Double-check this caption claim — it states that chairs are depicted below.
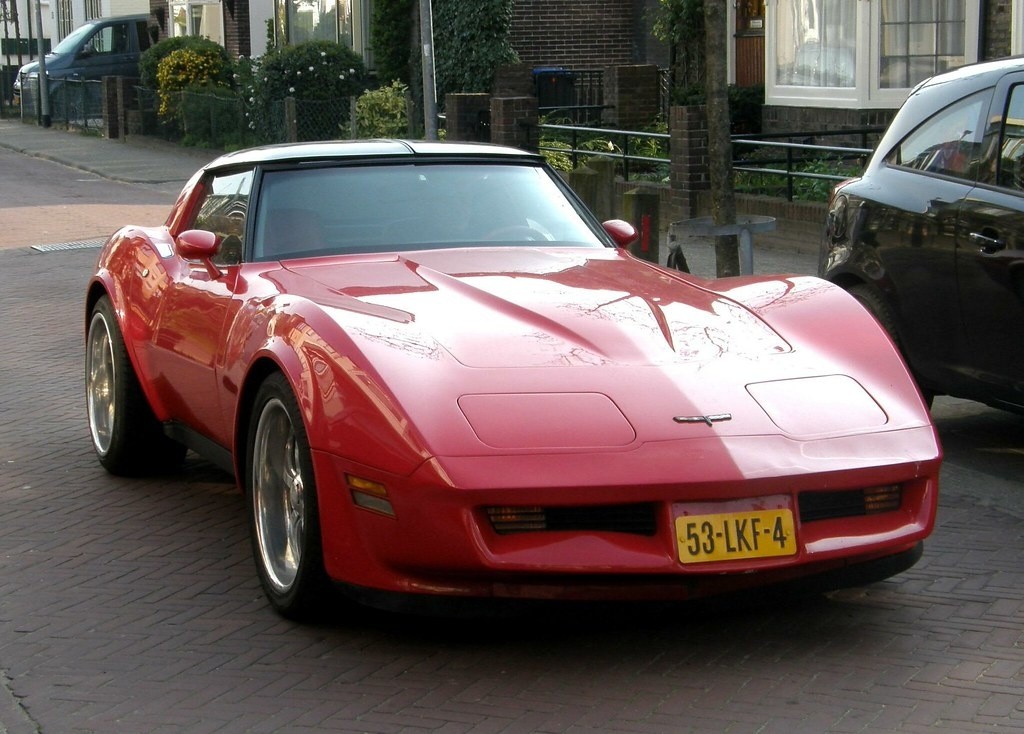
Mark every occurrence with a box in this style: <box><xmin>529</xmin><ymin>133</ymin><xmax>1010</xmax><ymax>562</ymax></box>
<box><xmin>431</xmin><ymin>178</ymin><xmax>530</xmax><ymax>240</ymax></box>
<box><xmin>263</xmin><ymin>208</ymin><xmax>324</xmax><ymax>252</ymax></box>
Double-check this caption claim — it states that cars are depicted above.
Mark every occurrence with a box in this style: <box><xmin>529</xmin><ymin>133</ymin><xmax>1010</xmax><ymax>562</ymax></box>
<box><xmin>817</xmin><ymin>54</ymin><xmax>1024</xmax><ymax>414</ymax></box>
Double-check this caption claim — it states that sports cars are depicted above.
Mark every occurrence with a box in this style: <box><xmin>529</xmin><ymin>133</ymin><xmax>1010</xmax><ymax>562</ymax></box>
<box><xmin>85</xmin><ymin>139</ymin><xmax>944</xmax><ymax>620</ymax></box>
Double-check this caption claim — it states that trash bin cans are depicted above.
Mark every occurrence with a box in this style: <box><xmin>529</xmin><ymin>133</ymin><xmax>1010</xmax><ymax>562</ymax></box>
<box><xmin>534</xmin><ymin>68</ymin><xmax>576</xmax><ymax>124</ymax></box>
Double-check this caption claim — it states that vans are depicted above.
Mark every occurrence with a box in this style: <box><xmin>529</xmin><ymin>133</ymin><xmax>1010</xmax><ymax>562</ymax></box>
<box><xmin>13</xmin><ymin>13</ymin><xmax>151</xmax><ymax>116</ymax></box>
